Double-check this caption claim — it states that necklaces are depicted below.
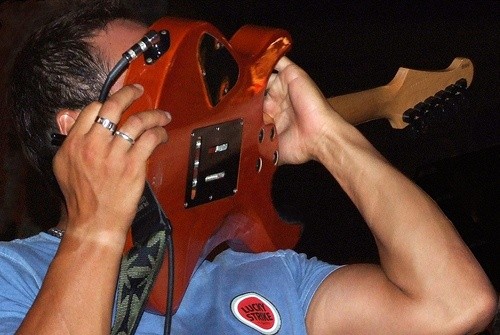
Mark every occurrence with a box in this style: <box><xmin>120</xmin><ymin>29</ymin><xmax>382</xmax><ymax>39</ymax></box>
<box><xmin>47</xmin><ymin>226</ymin><xmax>66</xmax><ymax>238</ymax></box>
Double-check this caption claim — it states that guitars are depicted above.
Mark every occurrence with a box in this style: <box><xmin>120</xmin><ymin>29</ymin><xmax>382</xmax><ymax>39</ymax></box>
<box><xmin>114</xmin><ymin>17</ymin><xmax>473</xmax><ymax>317</ymax></box>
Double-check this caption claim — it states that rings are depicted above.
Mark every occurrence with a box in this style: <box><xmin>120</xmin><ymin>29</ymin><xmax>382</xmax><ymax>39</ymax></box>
<box><xmin>95</xmin><ymin>116</ymin><xmax>117</xmax><ymax>133</ymax></box>
<box><xmin>114</xmin><ymin>130</ymin><xmax>135</xmax><ymax>145</ymax></box>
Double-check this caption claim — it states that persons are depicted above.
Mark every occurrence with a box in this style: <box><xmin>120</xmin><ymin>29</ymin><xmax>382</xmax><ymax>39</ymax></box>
<box><xmin>0</xmin><ymin>5</ymin><xmax>500</xmax><ymax>335</ymax></box>
